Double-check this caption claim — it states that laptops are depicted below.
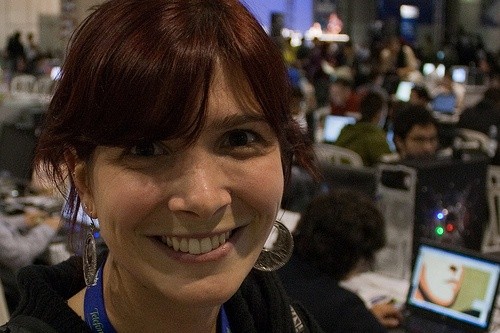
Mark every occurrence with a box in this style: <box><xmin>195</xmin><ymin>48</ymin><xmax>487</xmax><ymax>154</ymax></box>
<box><xmin>49</xmin><ymin>186</ymin><xmax>101</xmax><ymax>244</ymax></box>
<box><xmin>321</xmin><ymin>115</ymin><xmax>356</xmax><ymax>144</ymax></box>
<box><xmin>385</xmin><ymin>237</ymin><xmax>500</xmax><ymax>333</ymax></box>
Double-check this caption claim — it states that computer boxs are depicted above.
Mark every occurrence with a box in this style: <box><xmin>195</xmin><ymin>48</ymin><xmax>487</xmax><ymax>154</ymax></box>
<box><xmin>371</xmin><ymin>153</ymin><xmax>488</xmax><ymax>279</ymax></box>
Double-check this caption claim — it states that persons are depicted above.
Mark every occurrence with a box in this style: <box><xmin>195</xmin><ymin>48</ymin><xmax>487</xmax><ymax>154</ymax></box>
<box><xmin>1</xmin><ymin>204</ymin><xmax>64</xmax><ymax>270</ymax></box>
<box><xmin>3</xmin><ymin>0</ymin><xmax>386</xmax><ymax>331</ymax></box>
<box><xmin>292</xmin><ymin>192</ymin><xmax>404</xmax><ymax>330</ymax></box>
<box><xmin>5</xmin><ymin>29</ymin><xmax>56</xmax><ymax>76</ymax></box>
<box><xmin>280</xmin><ymin>32</ymin><xmax>499</xmax><ymax>166</ymax></box>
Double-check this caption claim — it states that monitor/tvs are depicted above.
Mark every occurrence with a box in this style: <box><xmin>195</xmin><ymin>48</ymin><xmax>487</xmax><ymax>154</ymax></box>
<box><xmin>449</xmin><ymin>65</ymin><xmax>469</xmax><ymax>85</ymax></box>
<box><xmin>395</xmin><ymin>81</ymin><xmax>416</xmax><ymax>103</ymax></box>
